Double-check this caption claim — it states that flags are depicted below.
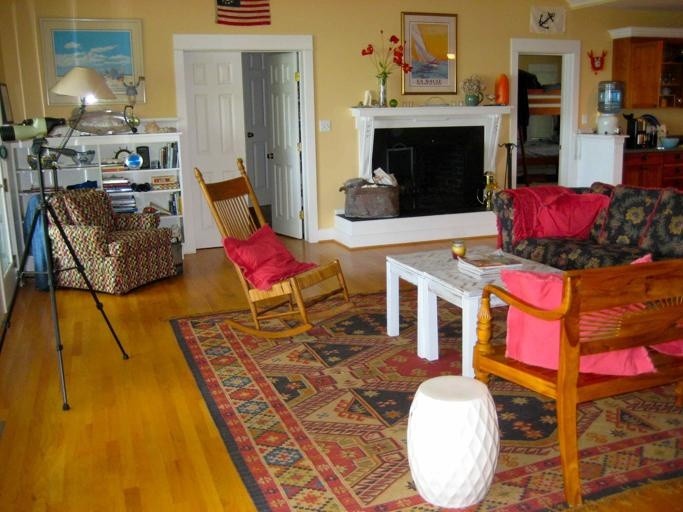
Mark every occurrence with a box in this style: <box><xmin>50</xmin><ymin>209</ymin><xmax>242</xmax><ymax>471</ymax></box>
<box><xmin>216</xmin><ymin>1</ymin><xmax>272</xmax><ymax>26</ymax></box>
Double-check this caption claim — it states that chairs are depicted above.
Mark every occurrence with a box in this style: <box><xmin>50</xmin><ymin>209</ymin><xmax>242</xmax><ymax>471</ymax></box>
<box><xmin>194</xmin><ymin>159</ymin><xmax>356</xmax><ymax>341</ymax></box>
<box><xmin>24</xmin><ymin>188</ymin><xmax>178</xmax><ymax>296</ymax></box>
<box><xmin>473</xmin><ymin>258</ymin><xmax>683</xmax><ymax>507</ymax></box>
<box><xmin>494</xmin><ymin>183</ymin><xmax>683</xmax><ymax>271</ymax></box>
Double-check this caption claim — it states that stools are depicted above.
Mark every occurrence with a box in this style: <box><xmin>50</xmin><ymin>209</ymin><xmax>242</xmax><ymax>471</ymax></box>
<box><xmin>406</xmin><ymin>375</ymin><xmax>500</xmax><ymax>511</ymax></box>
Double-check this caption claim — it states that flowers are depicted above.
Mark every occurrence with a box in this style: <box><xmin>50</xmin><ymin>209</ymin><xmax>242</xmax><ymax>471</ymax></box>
<box><xmin>361</xmin><ymin>28</ymin><xmax>413</xmax><ymax>85</ymax></box>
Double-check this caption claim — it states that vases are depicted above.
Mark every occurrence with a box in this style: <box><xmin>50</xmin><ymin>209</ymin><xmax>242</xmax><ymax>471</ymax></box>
<box><xmin>379</xmin><ymin>72</ymin><xmax>386</xmax><ymax>106</ymax></box>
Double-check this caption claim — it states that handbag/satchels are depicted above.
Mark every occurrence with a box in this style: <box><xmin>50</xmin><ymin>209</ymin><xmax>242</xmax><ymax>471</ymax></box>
<box><xmin>341</xmin><ymin>176</ymin><xmax>401</xmax><ymax>220</ymax></box>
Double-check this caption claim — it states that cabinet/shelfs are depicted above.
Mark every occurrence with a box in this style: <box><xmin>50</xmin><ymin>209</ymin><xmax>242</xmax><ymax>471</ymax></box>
<box><xmin>612</xmin><ymin>36</ymin><xmax>662</xmax><ymax>109</ymax></box>
<box><xmin>659</xmin><ymin>38</ymin><xmax>683</xmax><ymax>109</ymax></box>
<box><xmin>10</xmin><ymin>132</ymin><xmax>186</xmax><ymax>273</ymax></box>
<box><xmin>623</xmin><ymin>151</ymin><xmax>683</xmax><ymax>190</ymax></box>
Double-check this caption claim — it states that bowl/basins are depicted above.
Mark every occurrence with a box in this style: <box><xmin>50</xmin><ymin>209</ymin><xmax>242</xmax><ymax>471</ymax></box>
<box><xmin>659</xmin><ymin>137</ymin><xmax>680</xmax><ymax>150</ymax></box>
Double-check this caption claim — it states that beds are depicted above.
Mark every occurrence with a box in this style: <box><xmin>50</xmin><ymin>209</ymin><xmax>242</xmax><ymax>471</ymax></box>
<box><xmin>517</xmin><ymin>87</ymin><xmax>561</xmax><ymax>183</ymax></box>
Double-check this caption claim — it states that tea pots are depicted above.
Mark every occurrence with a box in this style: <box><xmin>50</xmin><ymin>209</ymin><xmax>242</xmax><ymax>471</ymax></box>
<box><xmin>465</xmin><ymin>92</ymin><xmax>483</xmax><ymax>106</ymax></box>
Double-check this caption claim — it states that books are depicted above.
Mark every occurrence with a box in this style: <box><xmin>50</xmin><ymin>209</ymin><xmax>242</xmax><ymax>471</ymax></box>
<box><xmin>456</xmin><ymin>247</ymin><xmax>523</xmax><ymax>283</ymax></box>
<box><xmin>101</xmin><ymin>158</ymin><xmax>139</xmax><ymax>214</ymax></box>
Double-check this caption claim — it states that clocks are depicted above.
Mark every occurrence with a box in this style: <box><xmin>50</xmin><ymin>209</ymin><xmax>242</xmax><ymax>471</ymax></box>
<box><xmin>111</xmin><ymin>145</ymin><xmax>133</xmax><ymax>166</ymax></box>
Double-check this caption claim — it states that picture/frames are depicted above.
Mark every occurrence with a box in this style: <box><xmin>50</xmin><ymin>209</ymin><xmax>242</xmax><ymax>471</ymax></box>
<box><xmin>40</xmin><ymin>17</ymin><xmax>146</xmax><ymax>104</ymax></box>
<box><xmin>401</xmin><ymin>12</ymin><xmax>457</xmax><ymax>95</ymax></box>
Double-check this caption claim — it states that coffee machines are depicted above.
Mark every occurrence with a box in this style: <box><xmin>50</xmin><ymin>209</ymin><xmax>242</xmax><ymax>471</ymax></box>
<box><xmin>624</xmin><ymin>110</ymin><xmax>658</xmax><ymax>151</ymax></box>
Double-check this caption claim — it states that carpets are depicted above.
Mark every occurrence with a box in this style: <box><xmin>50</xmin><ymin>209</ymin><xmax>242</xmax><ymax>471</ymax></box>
<box><xmin>170</xmin><ymin>287</ymin><xmax>681</xmax><ymax>512</ymax></box>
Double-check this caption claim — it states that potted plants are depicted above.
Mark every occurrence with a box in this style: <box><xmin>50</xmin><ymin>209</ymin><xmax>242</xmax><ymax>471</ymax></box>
<box><xmin>459</xmin><ymin>72</ymin><xmax>487</xmax><ymax>106</ymax></box>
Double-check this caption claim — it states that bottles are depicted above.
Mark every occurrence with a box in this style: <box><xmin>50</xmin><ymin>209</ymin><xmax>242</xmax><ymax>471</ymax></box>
<box><xmin>495</xmin><ymin>73</ymin><xmax>509</xmax><ymax>104</ymax></box>
<box><xmin>451</xmin><ymin>240</ymin><xmax>466</xmax><ymax>259</ymax></box>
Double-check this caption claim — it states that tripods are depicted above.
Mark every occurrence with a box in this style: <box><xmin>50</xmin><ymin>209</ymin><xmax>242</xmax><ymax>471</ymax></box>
<box><xmin>0</xmin><ymin>144</ymin><xmax>130</xmax><ymax>412</ymax></box>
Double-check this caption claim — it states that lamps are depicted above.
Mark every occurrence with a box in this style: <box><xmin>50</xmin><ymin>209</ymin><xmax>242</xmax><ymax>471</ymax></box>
<box><xmin>44</xmin><ymin>68</ymin><xmax>117</xmax><ymax>192</ymax></box>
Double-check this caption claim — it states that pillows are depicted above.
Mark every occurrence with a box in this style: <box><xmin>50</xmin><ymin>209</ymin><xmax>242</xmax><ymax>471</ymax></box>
<box><xmin>221</xmin><ymin>228</ymin><xmax>319</xmax><ymax>291</ymax></box>
<box><xmin>499</xmin><ymin>253</ymin><xmax>683</xmax><ymax>376</ymax></box>
<box><xmin>521</xmin><ymin>183</ymin><xmax>683</xmax><ymax>258</ymax></box>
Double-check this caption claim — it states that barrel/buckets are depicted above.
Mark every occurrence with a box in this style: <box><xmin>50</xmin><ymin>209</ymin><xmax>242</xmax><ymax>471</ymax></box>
<box><xmin>597</xmin><ymin>80</ymin><xmax>623</xmax><ymax>114</ymax></box>
